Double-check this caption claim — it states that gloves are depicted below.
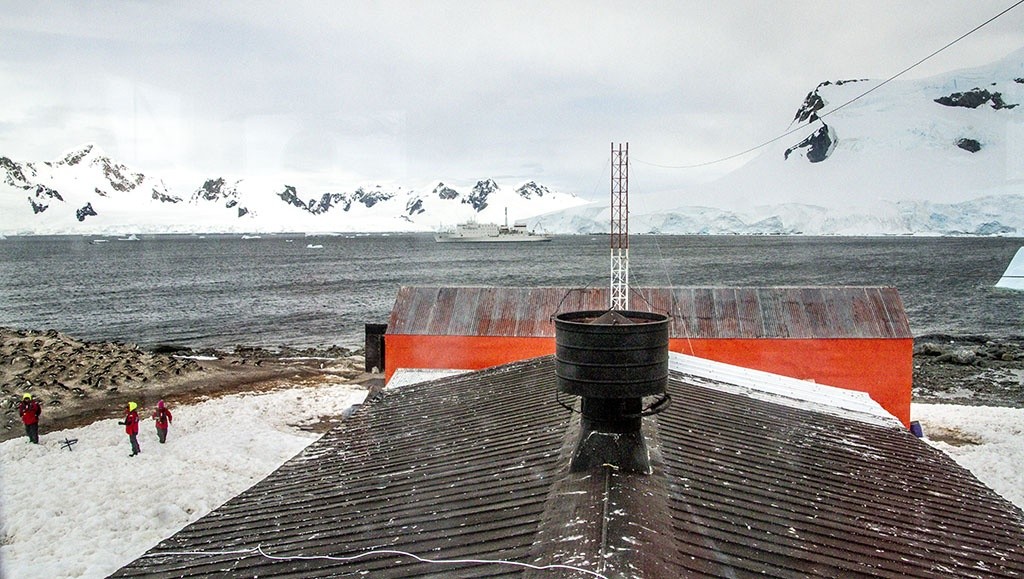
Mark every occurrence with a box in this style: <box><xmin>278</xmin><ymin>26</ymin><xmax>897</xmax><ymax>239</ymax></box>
<box><xmin>118</xmin><ymin>422</ymin><xmax>123</xmax><ymax>426</ymax></box>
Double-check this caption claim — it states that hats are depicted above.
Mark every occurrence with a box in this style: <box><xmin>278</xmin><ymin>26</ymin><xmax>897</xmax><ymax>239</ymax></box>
<box><xmin>159</xmin><ymin>400</ymin><xmax>164</xmax><ymax>408</ymax></box>
<box><xmin>128</xmin><ymin>402</ymin><xmax>137</xmax><ymax>411</ymax></box>
<box><xmin>22</xmin><ymin>393</ymin><xmax>31</xmax><ymax>400</ymax></box>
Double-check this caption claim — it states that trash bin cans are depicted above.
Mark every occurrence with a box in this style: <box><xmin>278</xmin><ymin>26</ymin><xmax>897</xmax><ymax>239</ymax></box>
<box><xmin>910</xmin><ymin>421</ymin><xmax>923</xmax><ymax>438</ymax></box>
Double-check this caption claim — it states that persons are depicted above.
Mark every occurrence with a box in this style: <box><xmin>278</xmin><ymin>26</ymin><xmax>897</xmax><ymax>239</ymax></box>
<box><xmin>152</xmin><ymin>401</ymin><xmax>173</xmax><ymax>443</ymax></box>
<box><xmin>18</xmin><ymin>393</ymin><xmax>42</xmax><ymax>444</ymax></box>
<box><xmin>118</xmin><ymin>402</ymin><xmax>141</xmax><ymax>457</ymax></box>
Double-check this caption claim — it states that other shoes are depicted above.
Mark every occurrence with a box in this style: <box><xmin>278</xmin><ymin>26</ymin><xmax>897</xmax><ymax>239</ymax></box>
<box><xmin>129</xmin><ymin>452</ymin><xmax>137</xmax><ymax>457</ymax></box>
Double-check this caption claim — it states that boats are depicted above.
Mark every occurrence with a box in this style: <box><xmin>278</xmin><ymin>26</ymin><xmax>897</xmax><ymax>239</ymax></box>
<box><xmin>435</xmin><ymin>205</ymin><xmax>552</xmax><ymax>243</ymax></box>
